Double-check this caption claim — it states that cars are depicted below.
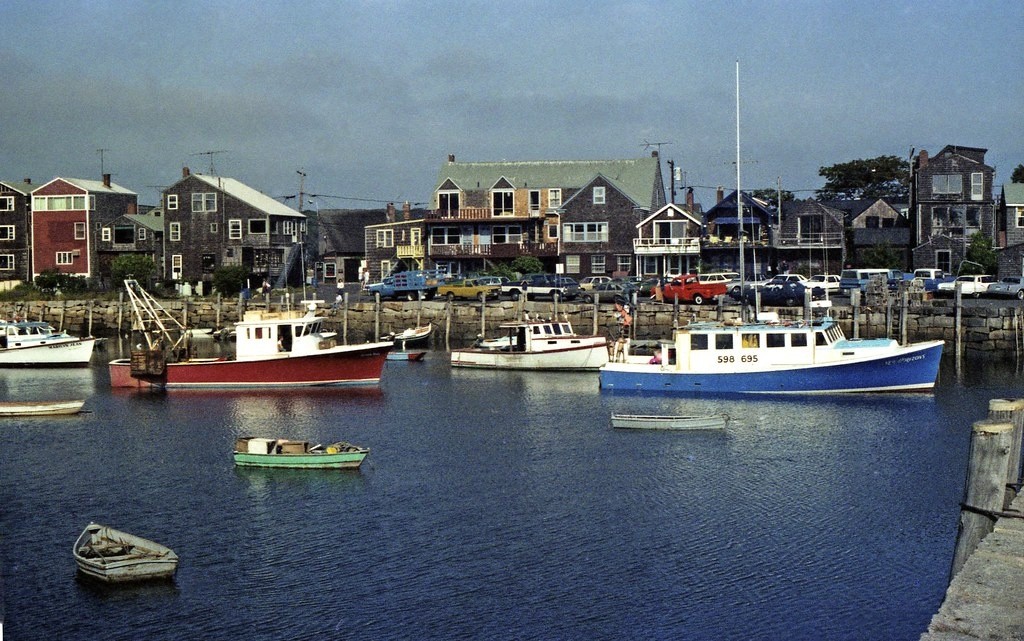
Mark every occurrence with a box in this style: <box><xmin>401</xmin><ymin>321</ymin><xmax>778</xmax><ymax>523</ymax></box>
<box><xmin>577</xmin><ymin>267</ymin><xmax>960</xmax><ymax>306</ymax></box>
<box><xmin>937</xmin><ymin>273</ymin><xmax>998</xmax><ymax>299</ymax></box>
<box><xmin>986</xmin><ymin>276</ymin><xmax>1024</xmax><ymax>301</ymax></box>
<box><xmin>581</xmin><ymin>280</ymin><xmax>643</xmax><ymax>305</ymax></box>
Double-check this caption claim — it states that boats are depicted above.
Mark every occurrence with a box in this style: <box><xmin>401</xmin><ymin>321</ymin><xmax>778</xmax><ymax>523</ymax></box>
<box><xmin>385</xmin><ymin>350</ymin><xmax>427</xmax><ymax>361</ymax></box>
<box><xmin>450</xmin><ymin>319</ymin><xmax>610</xmax><ymax>372</ymax></box>
<box><xmin>597</xmin><ymin>55</ymin><xmax>948</xmax><ymax>397</ymax></box>
<box><xmin>108</xmin><ymin>271</ymin><xmax>396</xmax><ymax>392</ymax></box>
<box><xmin>232</xmin><ymin>436</ymin><xmax>370</xmax><ymax>469</ymax></box>
<box><xmin>70</xmin><ymin>519</ymin><xmax>179</xmax><ymax>586</ymax></box>
<box><xmin>610</xmin><ymin>410</ymin><xmax>731</xmax><ymax>430</ymax></box>
<box><xmin>0</xmin><ymin>397</ymin><xmax>87</xmax><ymax>417</ymax></box>
<box><xmin>379</xmin><ymin>321</ymin><xmax>433</xmax><ymax>346</ymax></box>
<box><xmin>0</xmin><ymin>317</ymin><xmax>107</xmax><ymax>369</ymax></box>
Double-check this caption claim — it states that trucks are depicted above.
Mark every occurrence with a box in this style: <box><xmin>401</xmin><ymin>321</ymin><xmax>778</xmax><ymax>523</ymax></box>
<box><xmin>368</xmin><ymin>268</ymin><xmax>445</xmax><ymax>301</ymax></box>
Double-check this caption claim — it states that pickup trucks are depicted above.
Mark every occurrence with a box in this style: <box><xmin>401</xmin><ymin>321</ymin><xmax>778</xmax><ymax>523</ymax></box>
<box><xmin>650</xmin><ymin>274</ymin><xmax>728</xmax><ymax>305</ymax></box>
<box><xmin>436</xmin><ymin>277</ymin><xmax>501</xmax><ymax>301</ymax></box>
<box><xmin>501</xmin><ymin>274</ymin><xmax>580</xmax><ymax>302</ymax></box>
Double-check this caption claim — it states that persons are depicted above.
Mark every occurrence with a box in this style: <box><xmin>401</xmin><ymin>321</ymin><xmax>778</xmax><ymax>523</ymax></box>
<box><xmin>657</xmin><ymin>277</ymin><xmax>664</xmax><ymax>291</ymax></box>
<box><xmin>361</xmin><ymin>269</ymin><xmax>369</xmax><ymax>289</ymax></box>
<box><xmin>650</xmin><ymin>352</ymin><xmax>660</xmax><ymax>364</ymax></box>
<box><xmin>337</xmin><ymin>279</ymin><xmax>344</xmax><ymax>295</ymax></box>
<box><xmin>262</xmin><ymin>277</ymin><xmax>270</xmax><ymax>293</ymax></box>
<box><xmin>278</xmin><ymin>335</ymin><xmax>283</xmax><ymax>351</ymax></box>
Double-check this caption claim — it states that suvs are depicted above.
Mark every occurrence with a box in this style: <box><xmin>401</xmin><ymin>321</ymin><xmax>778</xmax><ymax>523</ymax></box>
<box><xmin>475</xmin><ymin>276</ymin><xmax>511</xmax><ymax>293</ymax></box>
